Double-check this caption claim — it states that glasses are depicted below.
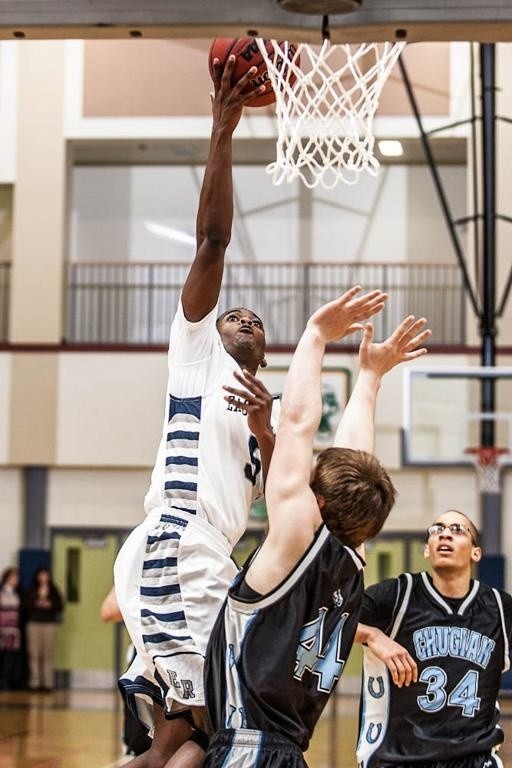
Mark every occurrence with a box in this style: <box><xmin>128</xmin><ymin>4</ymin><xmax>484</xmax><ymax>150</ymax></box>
<box><xmin>427</xmin><ymin>524</ymin><xmax>477</xmax><ymax>548</ymax></box>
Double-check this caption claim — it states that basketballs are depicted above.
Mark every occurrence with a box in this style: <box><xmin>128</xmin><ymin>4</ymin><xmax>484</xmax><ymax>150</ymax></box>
<box><xmin>209</xmin><ymin>37</ymin><xmax>300</xmax><ymax>107</ymax></box>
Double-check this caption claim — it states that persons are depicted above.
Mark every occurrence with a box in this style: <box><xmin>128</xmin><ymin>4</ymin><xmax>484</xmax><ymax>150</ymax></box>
<box><xmin>0</xmin><ymin>566</ymin><xmax>63</xmax><ymax>692</ymax></box>
<box><xmin>113</xmin><ymin>54</ymin><xmax>275</xmax><ymax>768</ymax></box>
<box><xmin>100</xmin><ymin>586</ymin><xmax>154</xmax><ymax>768</ymax></box>
<box><xmin>203</xmin><ymin>284</ymin><xmax>432</xmax><ymax>768</ymax></box>
<box><xmin>353</xmin><ymin>510</ymin><xmax>512</xmax><ymax>768</ymax></box>
